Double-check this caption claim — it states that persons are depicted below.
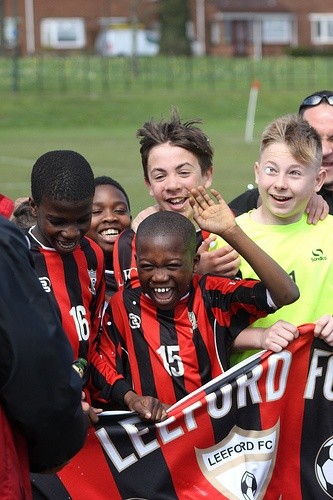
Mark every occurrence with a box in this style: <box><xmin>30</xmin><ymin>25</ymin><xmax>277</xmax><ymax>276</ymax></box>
<box><xmin>208</xmin><ymin>116</ymin><xmax>333</xmax><ymax>368</ymax></box>
<box><xmin>18</xmin><ymin>150</ymin><xmax>168</xmax><ymax>500</ymax></box>
<box><xmin>0</xmin><ymin>213</ymin><xmax>87</xmax><ymax>500</ymax></box>
<box><xmin>0</xmin><ymin>193</ymin><xmax>38</xmax><ymax>229</ymax></box>
<box><xmin>227</xmin><ymin>90</ymin><xmax>333</xmax><ymax>217</ymax></box>
<box><xmin>132</xmin><ymin>206</ymin><xmax>161</xmax><ymax>234</ymax></box>
<box><xmin>87</xmin><ymin>176</ymin><xmax>132</xmax><ymax>350</ymax></box>
<box><xmin>113</xmin><ymin>106</ymin><xmax>328</xmax><ymax>290</ymax></box>
<box><xmin>100</xmin><ymin>186</ymin><xmax>300</xmax><ymax>406</ymax></box>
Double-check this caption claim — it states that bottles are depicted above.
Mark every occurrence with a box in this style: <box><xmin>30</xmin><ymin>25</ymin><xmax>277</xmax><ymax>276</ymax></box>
<box><xmin>72</xmin><ymin>357</ymin><xmax>89</xmax><ymax>379</ymax></box>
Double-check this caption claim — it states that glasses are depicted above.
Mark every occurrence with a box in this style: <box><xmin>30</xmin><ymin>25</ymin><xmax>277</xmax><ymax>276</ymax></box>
<box><xmin>301</xmin><ymin>94</ymin><xmax>333</xmax><ymax>107</ymax></box>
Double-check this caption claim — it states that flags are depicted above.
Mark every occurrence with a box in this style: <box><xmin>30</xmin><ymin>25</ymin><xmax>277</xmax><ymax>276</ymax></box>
<box><xmin>30</xmin><ymin>323</ymin><xmax>333</xmax><ymax>500</ymax></box>
